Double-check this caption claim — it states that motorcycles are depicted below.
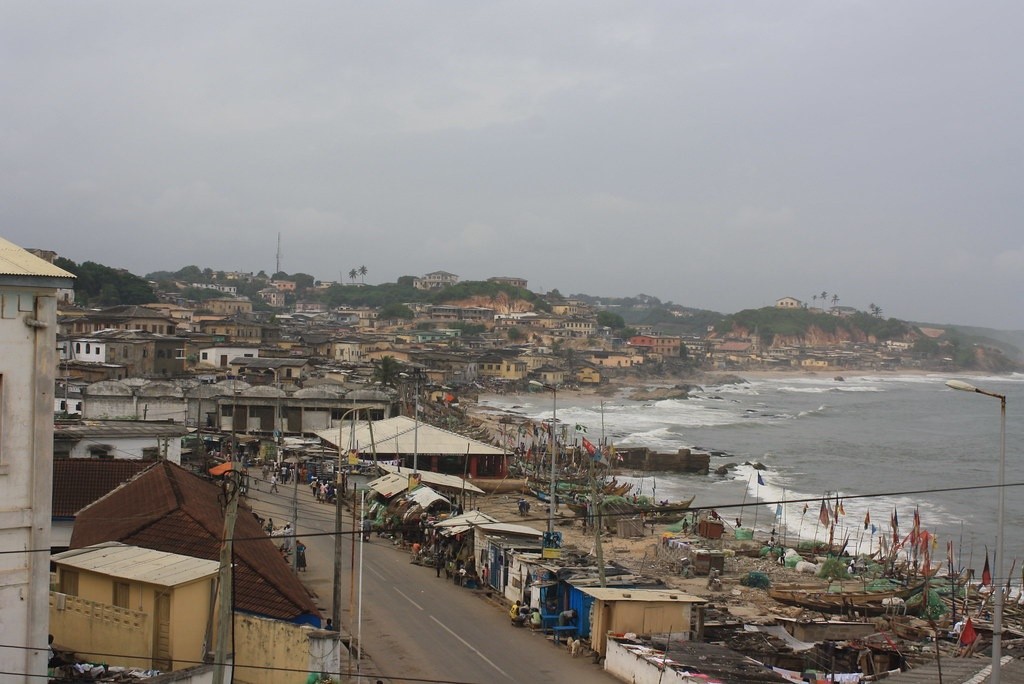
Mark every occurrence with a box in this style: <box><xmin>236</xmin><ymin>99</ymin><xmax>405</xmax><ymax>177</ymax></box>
<box><xmin>316</xmin><ymin>491</ymin><xmax>327</xmax><ymax>504</ymax></box>
<box><xmin>358</xmin><ymin>525</ymin><xmax>371</xmax><ymax>543</ymax></box>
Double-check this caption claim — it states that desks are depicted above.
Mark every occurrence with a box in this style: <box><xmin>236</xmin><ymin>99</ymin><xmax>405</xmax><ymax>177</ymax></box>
<box><xmin>553</xmin><ymin>625</ymin><xmax>579</xmax><ymax>644</ymax></box>
<box><xmin>454</xmin><ymin>571</ymin><xmax>474</xmax><ymax>586</ymax></box>
<box><xmin>541</xmin><ymin>615</ymin><xmax>560</xmax><ymax>634</ymax></box>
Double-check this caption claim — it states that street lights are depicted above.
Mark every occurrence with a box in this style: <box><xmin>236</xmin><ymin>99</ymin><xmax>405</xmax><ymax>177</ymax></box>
<box><xmin>942</xmin><ymin>378</ymin><xmax>1007</xmax><ymax>683</ymax></box>
<box><xmin>399</xmin><ymin>372</ymin><xmax>418</xmax><ymax>475</ymax></box>
<box><xmin>269</xmin><ymin>367</ymin><xmax>279</xmax><ymax>438</ymax></box>
<box><xmin>333</xmin><ymin>405</ymin><xmax>374</xmax><ymax>630</ymax></box>
<box><xmin>232</xmin><ymin>370</ymin><xmax>254</xmax><ymax>471</ymax></box>
<box><xmin>529</xmin><ymin>379</ymin><xmax>557</xmax><ymax>532</ymax></box>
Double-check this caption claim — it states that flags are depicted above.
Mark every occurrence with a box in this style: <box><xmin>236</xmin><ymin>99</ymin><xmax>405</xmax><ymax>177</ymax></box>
<box><xmin>758</xmin><ymin>474</ymin><xmax>991</xmax><ymax>644</ymax></box>
<box><xmin>510</xmin><ymin>422</ymin><xmax>624</xmax><ymax>467</ymax></box>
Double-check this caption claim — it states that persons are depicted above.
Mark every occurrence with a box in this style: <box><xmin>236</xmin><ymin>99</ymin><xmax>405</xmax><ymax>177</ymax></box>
<box><xmin>210</xmin><ymin>449</ymin><xmax>390</xmax><ymax>571</ymax></box>
<box><xmin>768</xmin><ymin>537</ymin><xmax>778</xmax><ymax>548</ymax></box>
<box><xmin>660</xmin><ymin>501</ymin><xmax>665</xmax><ymax>506</ymax></box>
<box><xmin>412</xmin><ymin>541</ymin><xmax>488</xmax><ymax>588</ymax></box>
<box><xmin>559</xmin><ymin>610</ymin><xmax>578</xmax><ymax>637</ymax></box>
<box><xmin>509</xmin><ymin>600</ymin><xmax>542</xmax><ymax>629</ymax></box>
<box><xmin>683</xmin><ymin>519</ymin><xmax>690</xmax><ymax>536</ymax></box>
<box><xmin>555</xmin><ymin>496</ymin><xmax>560</xmax><ymax>513</ymax></box>
<box><xmin>633</xmin><ymin>493</ymin><xmax>637</xmax><ymax>503</ymax></box>
<box><xmin>850</xmin><ymin>558</ymin><xmax>856</xmax><ymax>569</ymax></box>
<box><xmin>516</xmin><ymin>498</ymin><xmax>530</xmax><ymax>516</ymax></box>
<box><xmin>582</xmin><ymin>519</ymin><xmax>587</xmax><ymax>535</ymax></box>
<box><xmin>735</xmin><ymin>518</ymin><xmax>742</xmax><ymax>528</ymax></box>
<box><xmin>780</xmin><ymin>547</ymin><xmax>787</xmax><ymax>566</ymax></box>
<box><xmin>847</xmin><ymin>565</ymin><xmax>854</xmax><ymax>574</ymax></box>
